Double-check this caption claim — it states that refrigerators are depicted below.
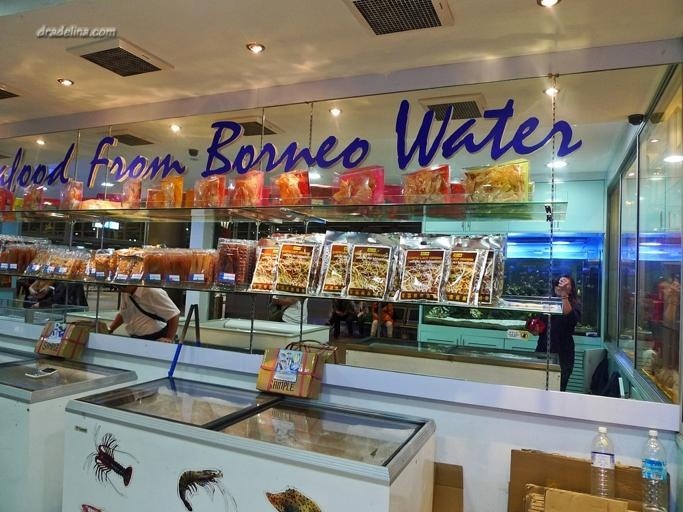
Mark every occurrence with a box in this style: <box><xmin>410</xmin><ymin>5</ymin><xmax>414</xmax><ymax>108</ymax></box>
<box><xmin>62</xmin><ymin>375</ymin><xmax>436</xmax><ymax>512</ymax></box>
<box><xmin>1</xmin><ymin>348</ymin><xmax>138</xmax><ymax>511</ymax></box>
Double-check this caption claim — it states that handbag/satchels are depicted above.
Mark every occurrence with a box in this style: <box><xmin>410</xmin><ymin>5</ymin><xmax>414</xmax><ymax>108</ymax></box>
<box><xmin>524</xmin><ymin>316</ymin><xmax>547</xmax><ymax>336</ymax></box>
<box><xmin>266</xmin><ymin>302</ymin><xmax>283</xmax><ymax>321</ymax></box>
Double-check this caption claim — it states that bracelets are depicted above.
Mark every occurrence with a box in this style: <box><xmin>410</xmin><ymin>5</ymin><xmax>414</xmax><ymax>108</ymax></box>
<box><xmin>107</xmin><ymin>326</ymin><xmax>113</xmax><ymax>333</ymax></box>
<box><xmin>562</xmin><ymin>295</ymin><xmax>569</xmax><ymax>299</ymax></box>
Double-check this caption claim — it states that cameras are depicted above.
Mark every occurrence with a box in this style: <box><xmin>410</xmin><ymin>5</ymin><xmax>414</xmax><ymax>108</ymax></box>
<box><xmin>551</xmin><ymin>280</ymin><xmax>559</xmax><ymax>287</ymax></box>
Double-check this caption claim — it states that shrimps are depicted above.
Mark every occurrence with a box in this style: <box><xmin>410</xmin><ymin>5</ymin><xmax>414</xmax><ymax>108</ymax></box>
<box><xmin>176</xmin><ymin>469</ymin><xmax>236</xmax><ymax>512</ymax></box>
<box><xmin>82</xmin><ymin>423</ymin><xmax>139</xmax><ymax>496</ymax></box>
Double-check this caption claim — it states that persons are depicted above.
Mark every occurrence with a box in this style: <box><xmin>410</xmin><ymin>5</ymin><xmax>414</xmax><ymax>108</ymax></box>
<box><xmin>16</xmin><ymin>275</ymin><xmax>88</xmax><ymax>308</ymax></box>
<box><xmin>325</xmin><ymin>299</ymin><xmax>394</xmax><ymax>338</ymax></box>
<box><xmin>271</xmin><ymin>294</ymin><xmax>309</xmax><ymax>324</ymax></box>
<box><xmin>108</xmin><ymin>286</ymin><xmax>181</xmax><ymax>343</ymax></box>
<box><xmin>528</xmin><ymin>275</ymin><xmax>581</xmax><ymax>391</ymax></box>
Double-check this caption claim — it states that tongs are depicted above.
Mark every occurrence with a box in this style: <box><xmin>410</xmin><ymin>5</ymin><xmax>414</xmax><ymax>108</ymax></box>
<box><xmin>168</xmin><ymin>304</ymin><xmax>201</xmax><ymax>379</ymax></box>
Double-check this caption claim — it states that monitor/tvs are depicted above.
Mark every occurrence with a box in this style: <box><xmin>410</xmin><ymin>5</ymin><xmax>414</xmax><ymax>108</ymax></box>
<box><xmin>393</xmin><ymin>306</ymin><xmax>407</xmax><ymax>322</ymax></box>
<box><xmin>407</xmin><ymin>308</ymin><xmax>420</xmax><ymax>324</ymax></box>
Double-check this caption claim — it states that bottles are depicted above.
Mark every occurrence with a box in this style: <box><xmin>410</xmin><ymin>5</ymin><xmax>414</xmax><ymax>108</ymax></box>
<box><xmin>590</xmin><ymin>426</ymin><xmax>615</xmax><ymax>500</ymax></box>
<box><xmin>640</xmin><ymin>430</ymin><xmax>668</xmax><ymax>510</ymax></box>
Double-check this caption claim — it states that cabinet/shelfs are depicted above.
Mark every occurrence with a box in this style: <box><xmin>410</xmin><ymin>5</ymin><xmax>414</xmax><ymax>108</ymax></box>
<box><xmin>0</xmin><ymin>201</ymin><xmax>569</xmax><ymax>315</ymax></box>
